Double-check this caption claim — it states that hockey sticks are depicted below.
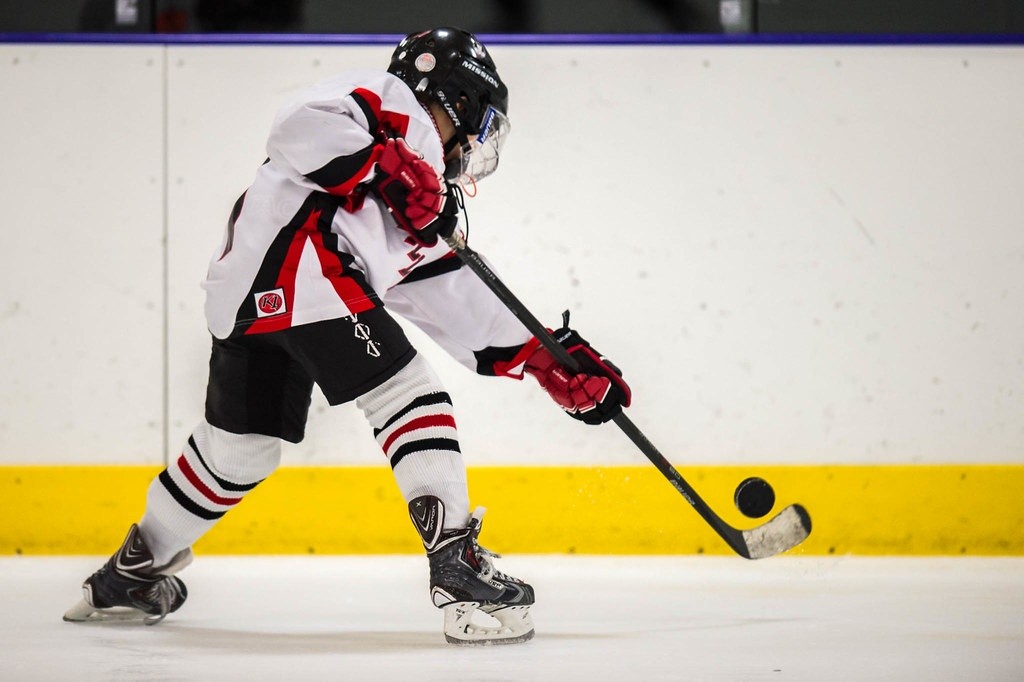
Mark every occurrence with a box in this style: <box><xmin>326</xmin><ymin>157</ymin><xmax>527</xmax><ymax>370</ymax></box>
<box><xmin>382</xmin><ymin>137</ymin><xmax>813</xmax><ymax>561</ymax></box>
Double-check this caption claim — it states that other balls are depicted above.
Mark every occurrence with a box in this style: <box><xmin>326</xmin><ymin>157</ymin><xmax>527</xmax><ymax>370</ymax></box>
<box><xmin>732</xmin><ymin>477</ymin><xmax>776</xmax><ymax>518</ymax></box>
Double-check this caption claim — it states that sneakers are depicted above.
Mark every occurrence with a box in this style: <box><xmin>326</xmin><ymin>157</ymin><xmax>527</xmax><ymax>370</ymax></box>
<box><xmin>63</xmin><ymin>523</ymin><xmax>193</xmax><ymax>626</ymax></box>
<box><xmin>408</xmin><ymin>495</ymin><xmax>535</xmax><ymax>646</ymax></box>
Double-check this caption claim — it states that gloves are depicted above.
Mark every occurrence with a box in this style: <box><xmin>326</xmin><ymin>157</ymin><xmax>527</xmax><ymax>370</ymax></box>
<box><xmin>523</xmin><ymin>310</ymin><xmax>631</xmax><ymax>425</ymax></box>
<box><xmin>362</xmin><ymin>137</ymin><xmax>459</xmax><ymax>248</ymax></box>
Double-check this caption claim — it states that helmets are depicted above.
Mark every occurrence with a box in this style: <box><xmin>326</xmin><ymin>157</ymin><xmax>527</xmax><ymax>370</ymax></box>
<box><xmin>387</xmin><ymin>26</ymin><xmax>510</xmax><ymax>186</ymax></box>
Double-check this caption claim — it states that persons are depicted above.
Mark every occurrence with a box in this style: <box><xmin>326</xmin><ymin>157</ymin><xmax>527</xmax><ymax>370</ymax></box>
<box><xmin>63</xmin><ymin>26</ymin><xmax>631</xmax><ymax>647</ymax></box>
